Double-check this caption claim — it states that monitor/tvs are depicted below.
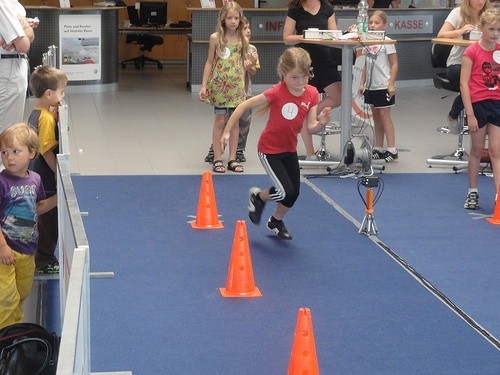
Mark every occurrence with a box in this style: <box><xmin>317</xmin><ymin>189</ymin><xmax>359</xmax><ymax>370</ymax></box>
<box><xmin>140</xmin><ymin>1</ymin><xmax>167</xmax><ymax>26</ymax></box>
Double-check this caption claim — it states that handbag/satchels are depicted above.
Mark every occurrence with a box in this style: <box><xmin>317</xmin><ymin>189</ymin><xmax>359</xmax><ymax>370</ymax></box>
<box><xmin>0</xmin><ymin>322</ymin><xmax>61</xmax><ymax>375</ymax></box>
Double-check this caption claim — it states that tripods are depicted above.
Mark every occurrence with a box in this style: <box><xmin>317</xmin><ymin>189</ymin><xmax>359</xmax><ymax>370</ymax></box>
<box><xmin>358</xmin><ymin>187</ymin><xmax>379</xmax><ymax>236</ymax></box>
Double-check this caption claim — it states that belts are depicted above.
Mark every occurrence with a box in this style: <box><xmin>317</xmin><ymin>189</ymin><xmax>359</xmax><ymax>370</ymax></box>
<box><xmin>1</xmin><ymin>53</ymin><xmax>25</xmax><ymax>59</ymax></box>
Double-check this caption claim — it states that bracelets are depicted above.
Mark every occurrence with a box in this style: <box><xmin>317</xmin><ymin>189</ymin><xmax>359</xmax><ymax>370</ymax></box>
<box><xmin>248</xmin><ymin>59</ymin><xmax>252</xmax><ymax>64</ymax></box>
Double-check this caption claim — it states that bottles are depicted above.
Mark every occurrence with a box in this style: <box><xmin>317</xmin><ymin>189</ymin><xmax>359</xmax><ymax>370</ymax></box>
<box><xmin>357</xmin><ymin>0</ymin><xmax>370</xmax><ymax>40</ymax></box>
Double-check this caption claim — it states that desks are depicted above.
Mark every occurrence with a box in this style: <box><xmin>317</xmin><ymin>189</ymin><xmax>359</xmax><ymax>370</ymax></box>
<box><xmin>293</xmin><ymin>36</ymin><xmax>397</xmax><ymax>171</ymax></box>
<box><xmin>118</xmin><ymin>24</ymin><xmax>193</xmax><ymax>36</ymax></box>
<box><xmin>429</xmin><ymin>35</ymin><xmax>492</xmax><ymax>174</ymax></box>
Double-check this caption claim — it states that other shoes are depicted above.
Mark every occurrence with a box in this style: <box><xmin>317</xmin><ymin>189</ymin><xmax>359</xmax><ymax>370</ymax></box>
<box><xmin>447</xmin><ymin>113</ymin><xmax>460</xmax><ymax>135</ymax></box>
<box><xmin>372</xmin><ymin>147</ymin><xmax>387</xmax><ymax>159</ymax></box>
<box><xmin>35</xmin><ymin>261</ymin><xmax>59</xmax><ymax>274</ymax></box>
<box><xmin>306</xmin><ymin>155</ymin><xmax>318</xmax><ymax>161</ymax></box>
<box><xmin>384</xmin><ymin>150</ymin><xmax>398</xmax><ymax>163</ymax></box>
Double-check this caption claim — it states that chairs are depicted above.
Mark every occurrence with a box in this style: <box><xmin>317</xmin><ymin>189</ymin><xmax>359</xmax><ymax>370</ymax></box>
<box><xmin>430</xmin><ymin>41</ymin><xmax>478</xmax><ymax>136</ymax></box>
<box><xmin>121</xmin><ymin>4</ymin><xmax>166</xmax><ymax>71</ymax></box>
<box><xmin>299</xmin><ymin>46</ymin><xmax>357</xmax><ymax>162</ymax></box>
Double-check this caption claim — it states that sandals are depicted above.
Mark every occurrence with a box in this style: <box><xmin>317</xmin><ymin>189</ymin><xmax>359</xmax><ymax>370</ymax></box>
<box><xmin>213</xmin><ymin>159</ymin><xmax>225</xmax><ymax>173</ymax></box>
<box><xmin>227</xmin><ymin>159</ymin><xmax>244</xmax><ymax>172</ymax></box>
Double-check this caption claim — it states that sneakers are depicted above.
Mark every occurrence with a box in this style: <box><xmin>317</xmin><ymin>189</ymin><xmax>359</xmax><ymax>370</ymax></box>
<box><xmin>265</xmin><ymin>215</ymin><xmax>292</xmax><ymax>240</ymax></box>
<box><xmin>205</xmin><ymin>144</ymin><xmax>214</xmax><ymax>162</ymax></box>
<box><xmin>464</xmin><ymin>191</ymin><xmax>480</xmax><ymax>210</ymax></box>
<box><xmin>236</xmin><ymin>149</ymin><xmax>246</xmax><ymax>162</ymax></box>
<box><xmin>248</xmin><ymin>186</ymin><xmax>266</xmax><ymax>224</ymax></box>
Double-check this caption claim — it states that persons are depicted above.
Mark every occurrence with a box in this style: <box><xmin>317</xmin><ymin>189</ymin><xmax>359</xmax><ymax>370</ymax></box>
<box><xmin>460</xmin><ymin>7</ymin><xmax>500</xmax><ymax>209</ymax></box>
<box><xmin>0</xmin><ymin>123</ymin><xmax>58</xmax><ymax>330</ymax></box>
<box><xmin>198</xmin><ymin>0</ymin><xmax>249</xmax><ymax>174</ymax></box>
<box><xmin>220</xmin><ymin>48</ymin><xmax>332</xmax><ymax>241</ymax></box>
<box><xmin>357</xmin><ymin>11</ymin><xmax>399</xmax><ymax>161</ymax></box>
<box><xmin>26</xmin><ymin>65</ymin><xmax>67</xmax><ymax>274</ymax></box>
<box><xmin>0</xmin><ymin>0</ymin><xmax>40</xmax><ymax>171</ymax></box>
<box><xmin>367</xmin><ymin>0</ymin><xmax>398</xmax><ymax>9</ymax></box>
<box><xmin>204</xmin><ymin>16</ymin><xmax>260</xmax><ymax>162</ymax></box>
<box><xmin>437</xmin><ymin>0</ymin><xmax>500</xmax><ymax>127</ymax></box>
<box><xmin>283</xmin><ymin>0</ymin><xmax>357</xmax><ymax>162</ymax></box>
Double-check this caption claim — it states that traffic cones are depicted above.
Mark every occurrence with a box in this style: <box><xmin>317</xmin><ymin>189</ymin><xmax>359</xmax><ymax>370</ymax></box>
<box><xmin>191</xmin><ymin>170</ymin><xmax>224</xmax><ymax>230</ymax></box>
<box><xmin>218</xmin><ymin>220</ymin><xmax>263</xmax><ymax>298</ymax></box>
<box><xmin>485</xmin><ymin>182</ymin><xmax>500</xmax><ymax>225</ymax></box>
<box><xmin>287</xmin><ymin>307</ymin><xmax>320</xmax><ymax>375</ymax></box>
